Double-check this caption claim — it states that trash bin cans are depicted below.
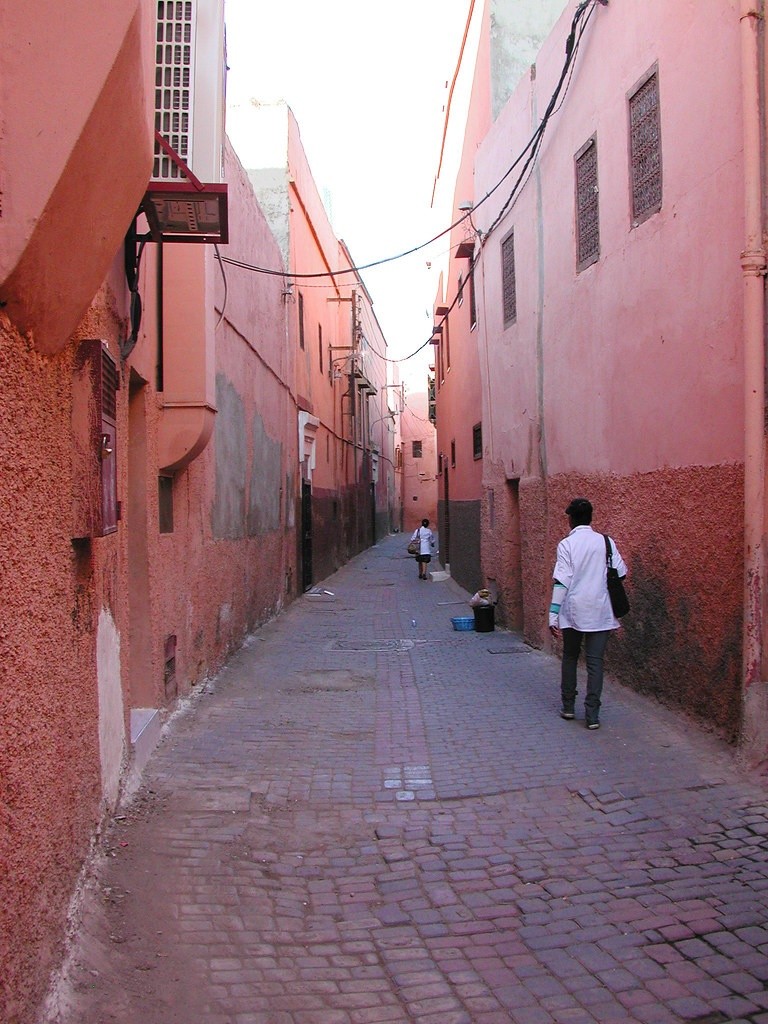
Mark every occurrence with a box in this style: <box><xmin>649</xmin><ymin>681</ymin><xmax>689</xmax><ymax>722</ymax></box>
<box><xmin>472</xmin><ymin>605</ymin><xmax>496</xmax><ymax>633</ymax></box>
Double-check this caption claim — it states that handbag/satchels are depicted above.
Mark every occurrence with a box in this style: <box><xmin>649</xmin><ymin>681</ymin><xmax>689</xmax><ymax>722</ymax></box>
<box><xmin>603</xmin><ymin>533</ymin><xmax>629</xmax><ymax>618</ymax></box>
<box><xmin>408</xmin><ymin>528</ymin><xmax>421</xmax><ymax>554</ymax></box>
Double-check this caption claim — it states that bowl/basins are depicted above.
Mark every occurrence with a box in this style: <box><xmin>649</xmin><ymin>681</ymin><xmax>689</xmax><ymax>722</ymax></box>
<box><xmin>450</xmin><ymin>617</ymin><xmax>475</xmax><ymax>631</ymax></box>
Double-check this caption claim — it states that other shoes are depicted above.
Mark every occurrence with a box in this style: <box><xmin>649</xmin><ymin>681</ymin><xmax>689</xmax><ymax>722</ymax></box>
<box><xmin>560</xmin><ymin>707</ymin><xmax>574</xmax><ymax>718</ymax></box>
<box><xmin>587</xmin><ymin>720</ymin><xmax>599</xmax><ymax>730</ymax></box>
<box><xmin>423</xmin><ymin>576</ymin><xmax>427</xmax><ymax>579</ymax></box>
<box><xmin>419</xmin><ymin>574</ymin><xmax>422</xmax><ymax>578</ymax></box>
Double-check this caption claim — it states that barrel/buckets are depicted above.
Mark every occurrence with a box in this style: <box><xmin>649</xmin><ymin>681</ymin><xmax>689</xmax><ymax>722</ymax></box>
<box><xmin>473</xmin><ymin>606</ymin><xmax>495</xmax><ymax>633</ymax></box>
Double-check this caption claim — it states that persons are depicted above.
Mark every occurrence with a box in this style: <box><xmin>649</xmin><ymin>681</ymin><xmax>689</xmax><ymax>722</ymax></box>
<box><xmin>548</xmin><ymin>498</ymin><xmax>628</xmax><ymax>729</ymax></box>
<box><xmin>409</xmin><ymin>519</ymin><xmax>435</xmax><ymax>580</ymax></box>
